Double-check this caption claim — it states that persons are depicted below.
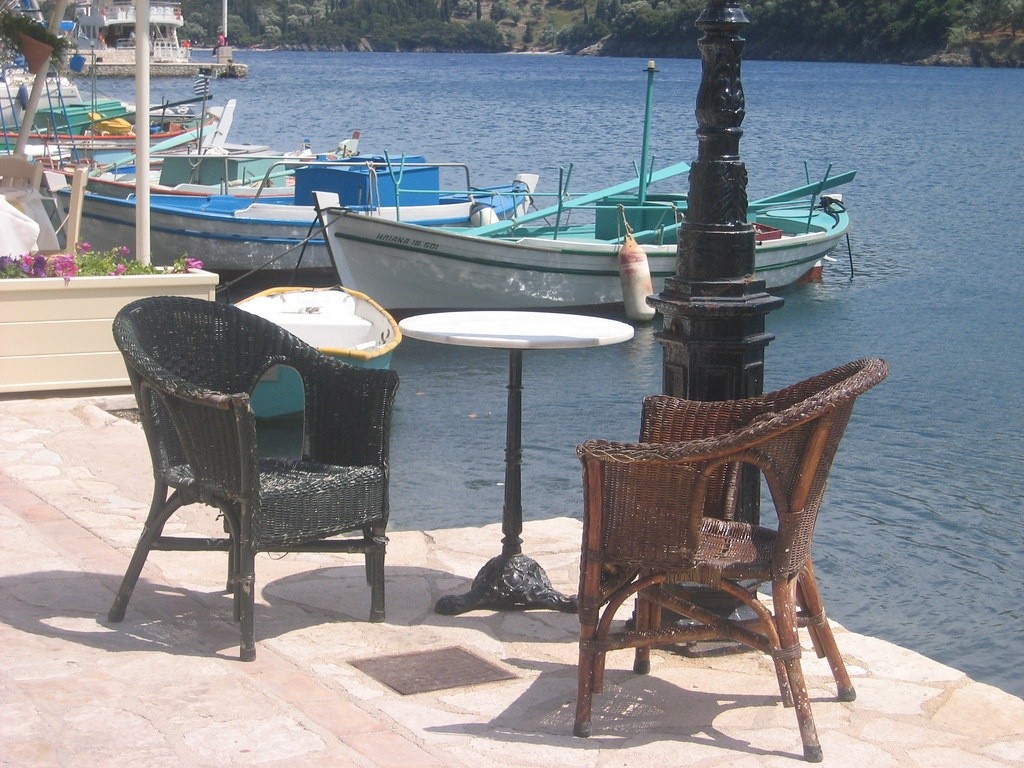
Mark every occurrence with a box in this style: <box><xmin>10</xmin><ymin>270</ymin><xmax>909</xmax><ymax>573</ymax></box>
<box><xmin>98</xmin><ymin>32</ymin><xmax>108</xmax><ymax>50</ymax></box>
<box><xmin>212</xmin><ymin>30</ymin><xmax>225</xmax><ymax>58</ymax></box>
<box><xmin>128</xmin><ymin>28</ymin><xmax>135</xmax><ymax>38</ymax></box>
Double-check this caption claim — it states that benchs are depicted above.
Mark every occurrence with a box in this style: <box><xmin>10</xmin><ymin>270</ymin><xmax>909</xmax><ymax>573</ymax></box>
<box><xmin>252</xmin><ymin>312</ymin><xmax>373</xmax><ymax>345</ymax></box>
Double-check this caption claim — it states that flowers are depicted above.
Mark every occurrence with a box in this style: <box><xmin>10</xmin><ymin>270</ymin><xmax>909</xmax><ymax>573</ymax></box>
<box><xmin>0</xmin><ymin>236</ymin><xmax>204</xmax><ymax>283</ymax></box>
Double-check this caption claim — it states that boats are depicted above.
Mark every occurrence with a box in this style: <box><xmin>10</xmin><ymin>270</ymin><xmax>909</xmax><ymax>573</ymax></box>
<box><xmin>1</xmin><ymin>0</ymin><xmax>859</xmax><ymax>325</ymax></box>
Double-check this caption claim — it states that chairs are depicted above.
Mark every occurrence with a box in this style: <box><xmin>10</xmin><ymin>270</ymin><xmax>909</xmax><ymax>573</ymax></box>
<box><xmin>107</xmin><ymin>295</ymin><xmax>399</xmax><ymax>662</ymax></box>
<box><xmin>573</xmin><ymin>357</ymin><xmax>887</xmax><ymax>763</ymax></box>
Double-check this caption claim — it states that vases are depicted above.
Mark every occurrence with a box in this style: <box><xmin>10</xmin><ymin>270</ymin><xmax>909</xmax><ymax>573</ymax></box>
<box><xmin>0</xmin><ymin>264</ymin><xmax>219</xmax><ymax>393</ymax></box>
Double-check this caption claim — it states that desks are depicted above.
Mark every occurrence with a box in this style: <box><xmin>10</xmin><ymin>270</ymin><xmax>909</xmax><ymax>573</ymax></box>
<box><xmin>399</xmin><ymin>311</ymin><xmax>634</xmax><ymax>616</ymax></box>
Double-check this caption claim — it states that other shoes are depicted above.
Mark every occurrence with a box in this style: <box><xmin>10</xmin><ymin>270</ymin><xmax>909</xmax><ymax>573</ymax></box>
<box><xmin>212</xmin><ymin>55</ymin><xmax>217</xmax><ymax>57</ymax></box>
